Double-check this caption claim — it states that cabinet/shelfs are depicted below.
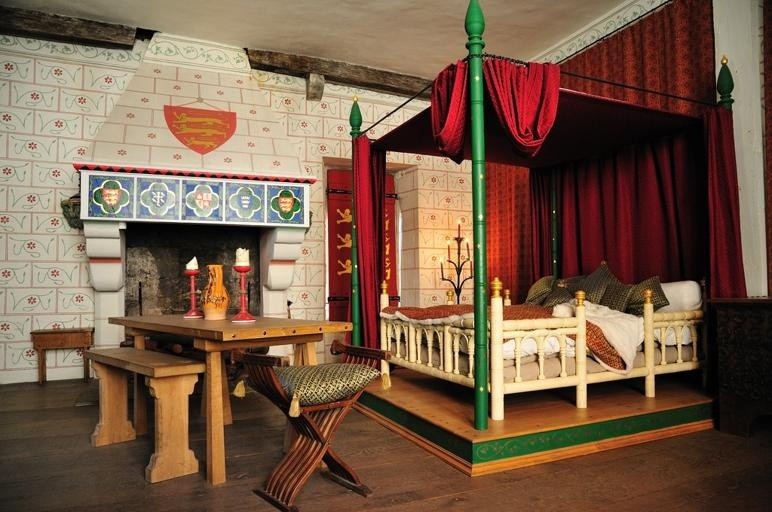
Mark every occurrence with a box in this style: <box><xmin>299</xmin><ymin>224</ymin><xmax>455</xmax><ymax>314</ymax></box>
<box><xmin>703</xmin><ymin>296</ymin><xmax>772</xmax><ymax>438</ymax></box>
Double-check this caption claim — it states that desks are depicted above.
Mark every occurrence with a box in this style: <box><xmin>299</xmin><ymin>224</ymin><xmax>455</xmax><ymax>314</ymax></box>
<box><xmin>29</xmin><ymin>326</ymin><xmax>94</xmax><ymax>386</ymax></box>
<box><xmin>108</xmin><ymin>313</ymin><xmax>354</xmax><ymax>487</ymax></box>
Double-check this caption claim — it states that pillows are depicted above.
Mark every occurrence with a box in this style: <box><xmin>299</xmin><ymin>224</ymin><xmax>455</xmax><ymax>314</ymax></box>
<box><xmin>525</xmin><ymin>261</ymin><xmax>702</xmax><ymax>317</ymax></box>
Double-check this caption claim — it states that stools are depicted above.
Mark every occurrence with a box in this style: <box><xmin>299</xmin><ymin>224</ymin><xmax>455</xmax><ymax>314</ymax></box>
<box><xmin>239</xmin><ymin>339</ymin><xmax>392</xmax><ymax>512</ymax></box>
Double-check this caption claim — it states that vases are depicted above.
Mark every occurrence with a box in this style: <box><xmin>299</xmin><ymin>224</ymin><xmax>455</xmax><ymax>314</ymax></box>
<box><xmin>200</xmin><ymin>264</ymin><xmax>231</xmax><ymax>321</ymax></box>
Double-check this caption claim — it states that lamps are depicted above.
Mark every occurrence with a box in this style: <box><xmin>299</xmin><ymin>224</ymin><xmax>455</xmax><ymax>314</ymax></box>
<box><xmin>440</xmin><ymin>221</ymin><xmax>474</xmax><ymax>304</ymax></box>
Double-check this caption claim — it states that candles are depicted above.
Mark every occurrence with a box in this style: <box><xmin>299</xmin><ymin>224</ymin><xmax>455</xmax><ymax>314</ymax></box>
<box><xmin>185</xmin><ymin>256</ymin><xmax>199</xmax><ymax>270</ymax></box>
<box><xmin>235</xmin><ymin>247</ymin><xmax>250</xmax><ymax>266</ymax></box>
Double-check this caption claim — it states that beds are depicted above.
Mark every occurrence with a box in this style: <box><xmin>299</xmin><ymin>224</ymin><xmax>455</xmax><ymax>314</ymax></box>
<box><xmin>379</xmin><ymin>274</ymin><xmax>708</xmax><ymax>421</ymax></box>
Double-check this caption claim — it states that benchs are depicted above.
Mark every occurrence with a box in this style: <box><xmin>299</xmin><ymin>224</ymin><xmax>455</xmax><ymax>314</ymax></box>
<box><xmin>83</xmin><ymin>347</ymin><xmax>207</xmax><ymax>484</ymax></box>
